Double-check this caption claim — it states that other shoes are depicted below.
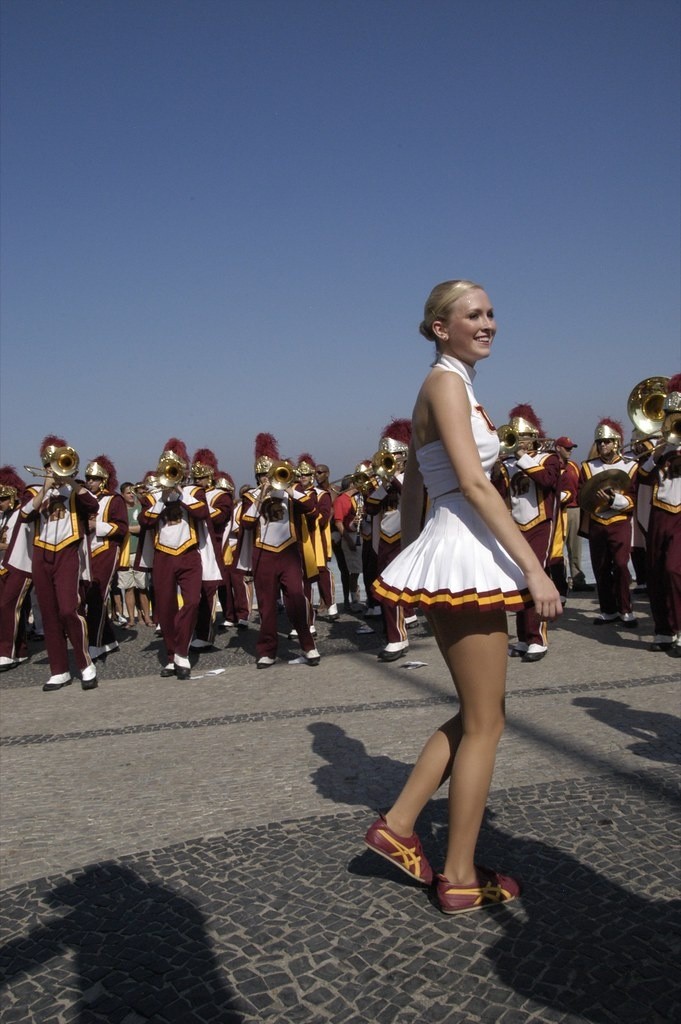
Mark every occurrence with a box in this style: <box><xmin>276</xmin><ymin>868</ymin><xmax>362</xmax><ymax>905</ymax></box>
<box><xmin>592</xmin><ymin>612</ymin><xmax>638</xmax><ymax>628</ymax></box>
<box><xmin>0</xmin><ymin>657</ymin><xmax>16</xmax><ymax>671</ymax></box>
<box><xmin>511</xmin><ymin>642</ymin><xmax>547</xmax><ymax>660</ymax></box>
<box><xmin>188</xmin><ymin>638</ymin><xmax>212</xmax><ymax>651</ymax></box>
<box><xmin>378</xmin><ymin>639</ymin><xmax>409</xmax><ymax>660</ymax></box>
<box><xmin>349</xmin><ymin>601</ymin><xmax>365</xmax><ymax>612</ymax></box>
<box><xmin>309</xmin><ymin>626</ymin><xmax>316</xmax><ymax>637</ymax></box>
<box><xmin>110</xmin><ymin>613</ymin><xmax>127</xmax><ymax>626</ymax></box>
<box><xmin>161</xmin><ymin>654</ymin><xmax>191</xmax><ymax>677</ymax></box>
<box><xmin>303</xmin><ymin>648</ymin><xmax>320</xmax><ymax>664</ymax></box>
<box><xmin>328</xmin><ymin>604</ymin><xmax>339</xmax><ymax>620</ymax></box>
<box><xmin>87</xmin><ymin>640</ymin><xmax>119</xmax><ymax>660</ymax></box>
<box><xmin>257</xmin><ymin>656</ymin><xmax>278</xmax><ymax>668</ymax></box>
<box><xmin>80</xmin><ymin>662</ymin><xmax>97</xmax><ymax>689</ymax></box>
<box><xmin>287</xmin><ymin>630</ymin><xmax>298</xmax><ymax>639</ymax></box>
<box><xmin>13</xmin><ymin>657</ymin><xmax>28</xmax><ymax>664</ymax></box>
<box><xmin>363</xmin><ymin>606</ymin><xmax>382</xmax><ymax>621</ymax></box>
<box><xmin>218</xmin><ymin>618</ymin><xmax>247</xmax><ymax>628</ymax></box>
<box><xmin>402</xmin><ymin>615</ymin><xmax>419</xmax><ymax>629</ymax></box>
<box><xmin>42</xmin><ymin>671</ymin><xmax>71</xmax><ymax>691</ymax></box>
<box><xmin>650</xmin><ymin>634</ymin><xmax>681</xmax><ymax>656</ymax></box>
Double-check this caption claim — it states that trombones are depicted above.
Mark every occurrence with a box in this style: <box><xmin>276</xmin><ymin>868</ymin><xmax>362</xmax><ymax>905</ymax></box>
<box><xmin>329</xmin><ymin>448</ymin><xmax>408</xmax><ymax>494</ymax></box>
<box><xmin>615</xmin><ymin>412</ymin><xmax>681</xmax><ymax>465</ymax></box>
<box><xmin>250</xmin><ymin>462</ymin><xmax>296</xmax><ymax>503</ymax></box>
<box><xmin>129</xmin><ymin>458</ymin><xmax>183</xmax><ymax>500</ymax></box>
<box><xmin>23</xmin><ymin>446</ymin><xmax>80</xmax><ymax>478</ymax></box>
<box><xmin>496</xmin><ymin>425</ymin><xmax>557</xmax><ymax>454</ymax></box>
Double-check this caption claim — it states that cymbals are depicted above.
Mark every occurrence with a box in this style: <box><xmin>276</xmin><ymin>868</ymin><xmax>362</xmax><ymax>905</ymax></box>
<box><xmin>577</xmin><ymin>468</ymin><xmax>633</xmax><ymax>515</ymax></box>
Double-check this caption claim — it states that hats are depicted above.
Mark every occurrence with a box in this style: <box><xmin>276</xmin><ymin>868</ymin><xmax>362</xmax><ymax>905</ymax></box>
<box><xmin>555</xmin><ymin>437</ymin><xmax>577</xmax><ymax>448</ymax></box>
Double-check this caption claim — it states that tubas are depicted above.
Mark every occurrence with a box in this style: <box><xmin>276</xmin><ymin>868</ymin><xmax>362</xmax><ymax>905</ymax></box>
<box><xmin>627</xmin><ymin>376</ymin><xmax>671</xmax><ymax>434</ymax></box>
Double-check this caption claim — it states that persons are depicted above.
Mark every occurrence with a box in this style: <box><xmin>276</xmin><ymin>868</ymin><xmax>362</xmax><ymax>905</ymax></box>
<box><xmin>362</xmin><ymin>278</ymin><xmax>561</xmax><ymax>916</ymax></box>
<box><xmin>0</xmin><ymin>418</ymin><xmax>418</xmax><ymax>692</ymax></box>
<box><xmin>488</xmin><ymin>375</ymin><xmax>681</xmax><ymax>662</ymax></box>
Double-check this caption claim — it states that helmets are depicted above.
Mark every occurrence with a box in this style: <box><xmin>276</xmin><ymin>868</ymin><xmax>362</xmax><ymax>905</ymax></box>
<box><xmin>190</xmin><ymin>449</ymin><xmax>235</xmax><ymax>497</ymax></box>
<box><xmin>663</xmin><ymin>374</ymin><xmax>681</xmax><ymax>411</ymax></box>
<box><xmin>0</xmin><ymin>466</ymin><xmax>26</xmax><ymax>509</ymax></box>
<box><xmin>509</xmin><ymin>404</ymin><xmax>545</xmax><ymax>450</ymax></box>
<box><xmin>160</xmin><ymin>438</ymin><xmax>189</xmax><ymax>477</ymax></box>
<box><xmin>594</xmin><ymin>418</ymin><xmax>623</xmax><ymax>456</ymax></box>
<box><xmin>379</xmin><ymin>420</ymin><xmax>411</xmax><ymax>473</ymax></box>
<box><xmin>254</xmin><ymin>433</ymin><xmax>278</xmax><ymax>473</ymax></box>
<box><xmin>296</xmin><ymin>454</ymin><xmax>314</xmax><ymax>483</ymax></box>
<box><xmin>85</xmin><ymin>455</ymin><xmax>118</xmax><ymax>492</ymax></box>
<box><xmin>40</xmin><ymin>434</ymin><xmax>67</xmax><ymax>466</ymax></box>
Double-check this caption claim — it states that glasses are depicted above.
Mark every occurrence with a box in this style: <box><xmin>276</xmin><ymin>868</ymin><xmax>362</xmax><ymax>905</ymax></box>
<box><xmin>317</xmin><ymin>470</ymin><xmax>327</xmax><ymax>475</ymax></box>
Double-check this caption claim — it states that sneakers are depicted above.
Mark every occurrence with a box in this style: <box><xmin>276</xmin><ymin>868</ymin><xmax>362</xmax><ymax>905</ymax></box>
<box><xmin>363</xmin><ymin>814</ymin><xmax>439</xmax><ymax>886</ymax></box>
<box><xmin>436</xmin><ymin>865</ymin><xmax>522</xmax><ymax>914</ymax></box>
